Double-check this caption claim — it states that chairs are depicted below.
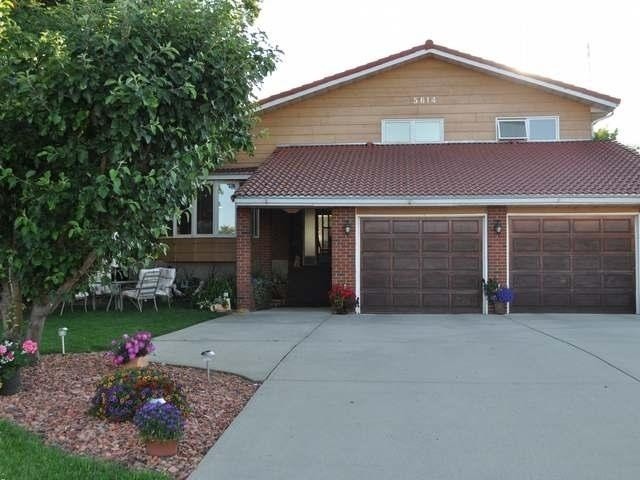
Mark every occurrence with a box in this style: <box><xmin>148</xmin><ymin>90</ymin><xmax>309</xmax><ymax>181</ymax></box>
<box><xmin>59</xmin><ymin>256</ymin><xmax>177</xmax><ymax>317</ymax></box>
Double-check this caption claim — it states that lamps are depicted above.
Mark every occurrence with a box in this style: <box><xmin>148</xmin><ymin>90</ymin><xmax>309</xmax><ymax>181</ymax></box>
<box><xmin>494</xmin><ymin>219</ymin><xmax>503</xmax><ymax>235</ymax></box>
<box><xmin>342</xmin><ymin>220</ymin><xmax>352</xmax><ymax>236</ymax></box>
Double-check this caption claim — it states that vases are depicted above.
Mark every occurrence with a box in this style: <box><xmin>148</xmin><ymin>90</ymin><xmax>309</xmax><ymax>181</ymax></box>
<box><xmin>144</xmin><ymin>437</ymin><xmax>177</xmax><ymax>456</ymax></box>
<box><xmin>494</xmin><ymin>302</ymin><xmax>506</xmax><ymax>314</ymax></box>
<box><xmin>0</xmin><ymin>367</ymin><xmax>20</xmax><ymax>396</ymax></box>
<box><xmin>332</xmin><ymin>299</ymin><xmax>349</xmax><ymax>314</ymax></box>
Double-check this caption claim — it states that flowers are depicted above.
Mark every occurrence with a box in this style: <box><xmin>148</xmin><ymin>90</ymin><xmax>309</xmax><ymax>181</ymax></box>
<box><xmin>0</xmin><ymin>337</ymin><xmax>39</xmax><ymax>387</ymax></box>
<box><xmin>327</xmin><ymin>285</ymin><xmax>356</xmax><ymax>306</ymax></box>
<box><xmin>484</xmin><ymin>280</ymin><xmax>514</xmax><ymax>304</ymax></box>
<box><xmin>89</xmin><ymin>329</ymin><xmax>195</xmax><ymax>445</ymax></box>
